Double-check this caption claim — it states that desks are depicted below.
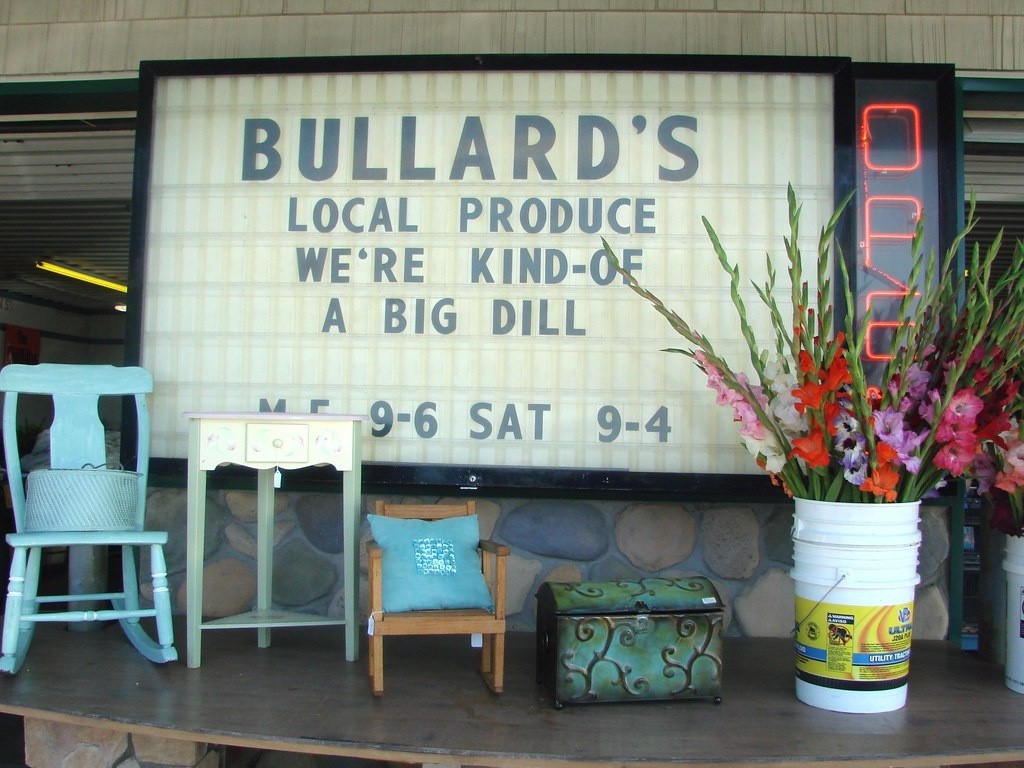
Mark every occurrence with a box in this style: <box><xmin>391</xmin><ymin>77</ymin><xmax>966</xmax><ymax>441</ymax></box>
<box><xmin>183</xmin><ymin>412</ymin><xmax>369</xmax><ymax>668</ymax></box>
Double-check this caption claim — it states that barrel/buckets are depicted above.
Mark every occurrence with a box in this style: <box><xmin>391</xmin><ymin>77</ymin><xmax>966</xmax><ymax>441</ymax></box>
<box><xmin>1002</xmin><ymin>534</ymin><xmax>1024</xmax><ymax>695</ymax></box>
<box><xmin>782</xmin><ymin>496</ymin><xmax>923</xmax><ymax>713</ymax></box>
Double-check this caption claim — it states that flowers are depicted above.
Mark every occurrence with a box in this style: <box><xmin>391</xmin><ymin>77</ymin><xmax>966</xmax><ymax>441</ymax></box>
<box><xmin>601</xmin><ymin>183</ymin><xmax>1024</xmax><ymax>539</ymax></box>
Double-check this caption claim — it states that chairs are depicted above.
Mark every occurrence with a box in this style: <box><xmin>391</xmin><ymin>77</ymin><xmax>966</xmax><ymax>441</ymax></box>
<box><xmin>0</xmin><ymin>363</ymin><xmax>178</xmax><ymax>676</ymax></box>
<box><xmin>365</xmin><ymin>501</ymin><xmax>510</xmax><ymax>696</ymax></box>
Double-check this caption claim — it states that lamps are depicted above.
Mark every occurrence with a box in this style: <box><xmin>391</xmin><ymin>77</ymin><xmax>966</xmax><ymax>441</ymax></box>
<box><xmin>32</xmin><ymin>256</ymin><xmax>128</xmax><ymax>294</ymax></box>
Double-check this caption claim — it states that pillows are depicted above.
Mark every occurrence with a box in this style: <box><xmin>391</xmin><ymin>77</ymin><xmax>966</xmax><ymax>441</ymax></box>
<box><xmin>366</xmin><ymin>511</ymin><xmax>496</xmax><ymax>616</ymax></box>
